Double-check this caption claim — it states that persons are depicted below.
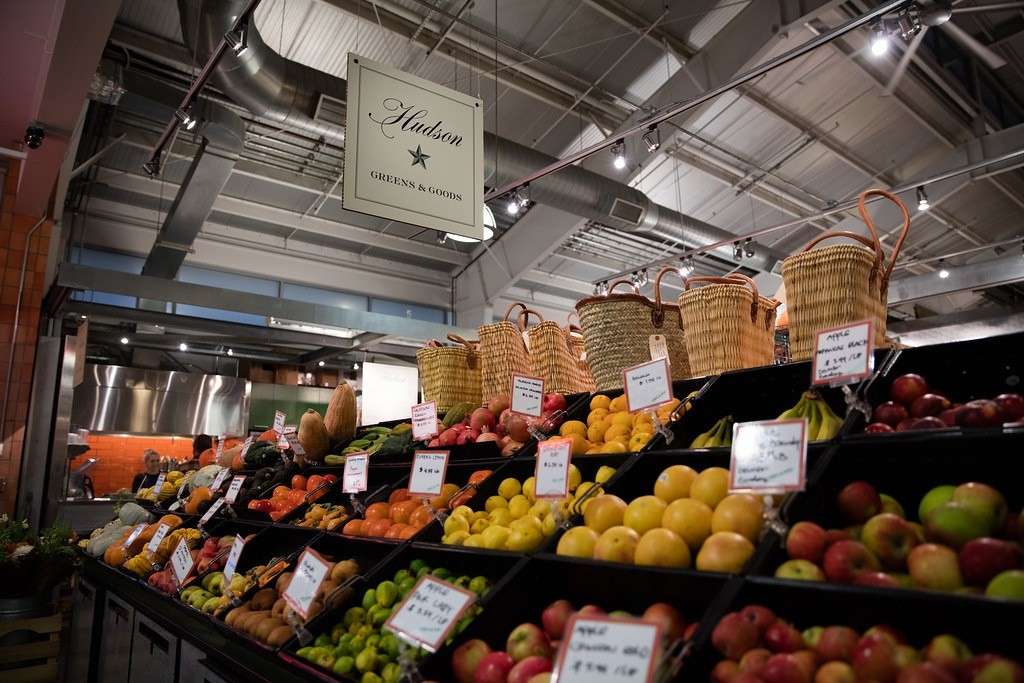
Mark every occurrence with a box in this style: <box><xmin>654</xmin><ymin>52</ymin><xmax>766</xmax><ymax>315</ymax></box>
<box><xmin>132</xmin><ymin>449</ymin><xmax>161</xmax><ymax>493</ymax></box>
<box><xmin>176</xmin><ymin>434</ymin><xmax>213</xmax><ymax>474</ymax></box>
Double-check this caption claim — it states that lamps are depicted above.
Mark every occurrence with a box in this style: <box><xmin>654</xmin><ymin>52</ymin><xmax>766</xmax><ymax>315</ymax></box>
<box><xmin>594</xmin><ymin>282</ymin><xmax>603</xmax><ymax>295</ymax></box>
<box><xmin>631</xmin><ymin>271</ymin><xmax>640</xmax><ymax>291</ymax></box>
<box><xmin>744</xmin><ymin>237</ymin><xmax>754</xmax><ymax>258</ymax></box>
<box><xmin>610</xmin><ymin>138</ymin><xmax>627</xmax><ymax>170</ymax></box>
<box><xmin>223</xmin><ymin>23</ymin><xmax>249</xmax><ymax>58</ymax></box>
<box><xmin>518</xmin><ymin>182</ymin><xmax>531</xmax><ymax>209</ymax></box>
<box><xmin>936</xmin><ymin>259</ymin><xmax>949</xmax><ymax>278</ymax></box>
<box><xmin>438</xmin><ymin>232</ymin><xmax>448</xmax><ymax>244</ymax></box>
<box><xmin>641</xmin><ymin>123</ymin><xmax>661</xmax><ymax>155</ymax></box>
<box><xmin>897</xmin><ymin>0</ymin><xmax>921</xmax><ymax>41</ymax></box>
<box><xmin>603</xmin><ymin>280</ymin><xmax>609</xmax><ymax>291</ymax></box>
<box><xmin>917</xmin><ymin>185</ymin><xmax>929</xmax><ymax>211</ymax></box>
<box><xmin>175</xmin><ymin>102</ymin><xmax>197</xmax><ymax>130</ymax></box>
<box><xmin>447</xmin><ymin>202</ymin><xmax>497</xmax><ymax>243</ymax></box>
<box><xmin>140</xmin><ymin>153</ymin><xmax>162</xmax><ymax>181</ymax></box>
<box><xmin>507</xmin><ymin>188</ymin><xmax>520</xmax><ymax>214</ymax></box>
<box><xmin>733</xmin><ymin>241</ymin><xmax>742</xmax><ymax>261</ymax></box>
<box><xmin>639</xmin><ymin>268</ymin><xmax>649</xmax><ymax>287</ymax></box>
<box><xmin>679</xmin><ymin>257</ymin><xmax>691</xmax><ymax>276</ymax></box>
<box><xmin>687</xmin><ymin>254</ymin><xmax>695</xmax><ymax>273</ymax></box>
<box><xmin>867</xmin><ymin>15</ymin><xmax>888</xmax><ymax>56</ymax></box>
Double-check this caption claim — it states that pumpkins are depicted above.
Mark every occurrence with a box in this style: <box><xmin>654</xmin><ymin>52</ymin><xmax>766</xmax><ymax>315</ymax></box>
<box><xmin>137</xmin><ymin>464</ymin><xmax>226</xmax><ymax>513</ymax></box>
<box><xmin>199</xmin><ymin>379</ymin><xmax>356</xmax><ymax>470</ymax></box>
<box><xmin>375</xmin><ymin>400</ymin><xmax>480</xmax><ymax>453</ymax></box>
<box><xmin>76</xmin><ymin>502</ymin><xmax>204</xmax><ymax>578</ymax></box>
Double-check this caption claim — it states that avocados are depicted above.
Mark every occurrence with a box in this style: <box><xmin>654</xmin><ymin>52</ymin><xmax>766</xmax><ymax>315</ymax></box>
<box><xmin>196</xmin><ymin>461</ymin><xmax>301</xmax><ymax>518</ymax></box>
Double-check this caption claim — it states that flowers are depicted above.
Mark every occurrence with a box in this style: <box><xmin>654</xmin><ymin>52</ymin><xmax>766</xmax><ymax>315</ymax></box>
<box><xmin>0</xmin><ymin>511</ymin><xmax>85</xmax><ymax>599</ymax></box>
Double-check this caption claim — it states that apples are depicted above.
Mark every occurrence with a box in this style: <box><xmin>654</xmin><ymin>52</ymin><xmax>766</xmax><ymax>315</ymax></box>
<box><xmin>147</xmin><ymin>534</ymin><xmax>257</xmax><ymax>594</ymax></box>
<box><xmin>858</xmin><ymin>374</ymin><xmax>1024</xmax><ymax>434</ymax></box>
<box><xmin>451</xmin><ymin>479</ymin><xmax>1023</xmax><ymax>683</ymax></box>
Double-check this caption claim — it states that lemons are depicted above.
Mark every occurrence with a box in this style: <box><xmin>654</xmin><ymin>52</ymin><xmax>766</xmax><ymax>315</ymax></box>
<box><xmin>440</xmin><ymin>463</ymin><xmax>617</xmax><ymax>553</ymax></box>
<box><xmin>292</xmin><ymin>560</ymin><xmax>489</xmax><ymax>683</ymax></box>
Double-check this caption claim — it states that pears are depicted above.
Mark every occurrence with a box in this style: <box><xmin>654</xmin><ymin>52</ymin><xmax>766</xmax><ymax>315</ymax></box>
<box><xmin>180</xmin><ymin>570</ymin><xmax>252</xmax><ymax>615</ymax></box>
<box><xmin>222</xmin><ymin>553</ymin><xmax>359</xmax><ymax>645</ymax></box>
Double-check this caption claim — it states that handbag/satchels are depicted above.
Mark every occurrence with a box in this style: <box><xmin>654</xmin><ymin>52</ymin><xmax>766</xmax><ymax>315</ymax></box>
<box><xmin>519</xmin><ymin>305</ymin><xmax>595</xmax><ymax>399</ymax></box>
<box><xmin>678</xmin><ymin>277</ymin><xmax>780</xmax><ymax>376</ymax></box>
<box><xmin>783</xmin><ymin>188</ymin><xmax>911</xmax><ymax>361</ymax></box>
<box><xmin>477</xmin><ymin>301</ymin><xmax>531</xmax><ymax>407</ymax></box>
<box><xmin>576</xmin><ymin>278</ymin><xmax>691</xmax><ymax>395</ymax></box>
<box><xmin>417</xmin><ymin>327</ymin><xmax>483</xmax><ymax>412</ymax></box>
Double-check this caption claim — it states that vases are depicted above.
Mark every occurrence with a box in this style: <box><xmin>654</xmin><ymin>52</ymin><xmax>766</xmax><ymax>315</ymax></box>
<box><xmin>0</xmin><ymin>595</ymin><xmax>43</xmax><ymax>672</ymax></box>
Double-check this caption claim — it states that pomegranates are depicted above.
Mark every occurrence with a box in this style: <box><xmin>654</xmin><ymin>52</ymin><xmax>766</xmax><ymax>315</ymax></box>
<box><xmin>428</xmin><ymin>390</ymin><xmax>567</xmax><ymax>456</ymax></box>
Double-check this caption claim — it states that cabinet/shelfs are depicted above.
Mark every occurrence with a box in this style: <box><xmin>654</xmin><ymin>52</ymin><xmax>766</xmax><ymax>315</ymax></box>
<box><xmin>63</xmin><ymin>572</ymin><xmax>237</xmax><ymax>683</ymax></box>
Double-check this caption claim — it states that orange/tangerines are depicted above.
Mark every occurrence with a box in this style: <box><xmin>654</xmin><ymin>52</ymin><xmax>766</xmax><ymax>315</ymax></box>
<box><xmin>545</xmin><ymin>390</ymin><xmax>700</xmax><ymax>453</ymax></box>
<box><xmin>554</xmin><ymin>466</ymin><xmax>782</xmax><ymax>577</ymax></box>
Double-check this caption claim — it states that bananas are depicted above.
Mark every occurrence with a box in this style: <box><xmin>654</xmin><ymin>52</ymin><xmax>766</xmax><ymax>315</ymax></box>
<box><xmin>688</xmin><ymin>390</ymin><xmax>845</xmax><ymax>449</ymax></box>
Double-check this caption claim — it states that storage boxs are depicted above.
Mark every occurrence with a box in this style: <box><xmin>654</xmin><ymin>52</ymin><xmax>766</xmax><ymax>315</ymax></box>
<box><xmin>0</xmin><ymin>607</ymin><xmax>62</xmax><ymax>683</ymax></box>
<box><xmin>273</xmin><ymin>365</ymin><xmax>298</xmax><ymax>386</ymax></box>
<box><xmin>70</xmin><ymin>326</ymin><xmax>1024</xmax><ymax>683</ymax></box>
<box><xmin>314</xmin><ymin>369</ymin><xmax>338</xmax><ymax>389</ymax></box>
<box><xmin>249</xmin><ymin>361</ymin><xmax>275</xmax><ymax>384</ymax></box>
